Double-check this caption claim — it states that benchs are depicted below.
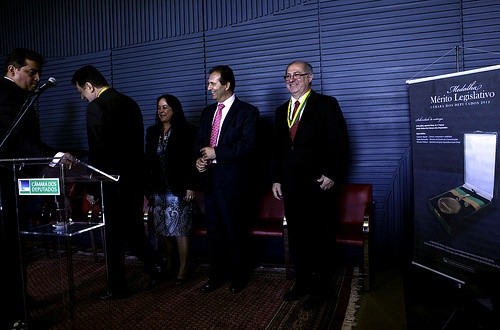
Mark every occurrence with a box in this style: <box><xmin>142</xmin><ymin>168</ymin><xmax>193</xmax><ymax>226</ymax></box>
<box><xmin>143</xmin><ymin>183</ymin><xmax>377</xmax><ymax>295</ymax></box>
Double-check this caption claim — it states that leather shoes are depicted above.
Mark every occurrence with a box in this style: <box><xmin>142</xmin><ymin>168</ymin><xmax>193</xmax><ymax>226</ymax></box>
<box><xmin>90</xmin><ymin>288</ymin><xmax>129</xmax><ymax>301</ymax></box>
<box><xmin>229</xmin><ymin>278</ymin><xmax>248</xmax><ymax>293</ymax></box>
<box><xmin>199</xmin><ymin>279</ymin><xmax>224</xmax><ymax>293</ymax></box>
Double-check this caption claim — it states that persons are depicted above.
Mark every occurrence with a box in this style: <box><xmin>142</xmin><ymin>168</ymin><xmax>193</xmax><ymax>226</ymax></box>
<box><xmin>191</xmin><ymin>65</ymin><xmax>261</xmax><ymax>293</ymax></box>
<box><xmin>145</xmin><ymin>94</ymin><xmax>195</xmax><ymax>286</ymax></box>
<box><xmin>0</xmin><ymin>47</ymin><xmax>74</xmax><ymax>330</ymax></box>
<box><xmin>71</xmin><ymin>64</ymin><xmax>144</xmax><ymax>300</ymax></box>
<box><xmin>272</xmin><ymin>61</ymin><xmax>352</xmax><ymax>309</ymax></box>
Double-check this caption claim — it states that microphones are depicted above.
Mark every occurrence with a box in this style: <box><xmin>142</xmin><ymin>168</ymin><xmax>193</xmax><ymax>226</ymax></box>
<box><xmin>28</xmin><ymin>77</ymin><xmax>57</xmax><ymax>98</ymax></box>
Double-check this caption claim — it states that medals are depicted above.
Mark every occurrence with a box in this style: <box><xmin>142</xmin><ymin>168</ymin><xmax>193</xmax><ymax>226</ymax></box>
<box><xmin>438</xmin><ymin>197</ymin><xmax>461</xmax><ymax>214</ymax></box>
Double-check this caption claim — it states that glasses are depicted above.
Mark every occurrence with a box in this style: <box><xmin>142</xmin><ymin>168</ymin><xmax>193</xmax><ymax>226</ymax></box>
<box><xmin>284</xmin><ymin>73</ymin><xmax>308</xmax><ymax>79</ymax></box>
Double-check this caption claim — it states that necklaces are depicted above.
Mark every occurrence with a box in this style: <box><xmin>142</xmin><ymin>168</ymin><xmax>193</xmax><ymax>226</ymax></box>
<box><xmin>287</xmin><ymin>89</ymin><xmax>312</xmax><ymax>129</ymax></box>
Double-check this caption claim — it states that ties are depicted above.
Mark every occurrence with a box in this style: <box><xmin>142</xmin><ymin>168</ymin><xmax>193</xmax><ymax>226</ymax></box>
<box><xmin>289</xmin><ymin>101</ymin><xmax>300</xmax><ymax>142</ymax></box>
<box><xmin>209</xmin><ymin>103</ymin><xmax>225</xmax><ymax>166</ymax></box>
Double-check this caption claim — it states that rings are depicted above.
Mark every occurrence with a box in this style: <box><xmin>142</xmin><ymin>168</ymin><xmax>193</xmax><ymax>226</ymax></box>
<box><xmin>189</xmin><ymin>195</ymin><xmax>194</xmax><ymax>200</ymax></box>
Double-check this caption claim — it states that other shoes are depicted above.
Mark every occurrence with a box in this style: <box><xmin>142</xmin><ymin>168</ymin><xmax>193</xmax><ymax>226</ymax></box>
<box><xmin>303</xmin><ymin>296</ymin><xmax>333</xmax><ymax>311</ymax></box>
<box><xmin>284</xmin><ymin>284</ymin><xmax>311</xmax><ymax>302</ymax></box>
<box><xmin>174</xmin><ymin>274</ymin><xmax>188</xmax><ymax>286</ymax></box>
